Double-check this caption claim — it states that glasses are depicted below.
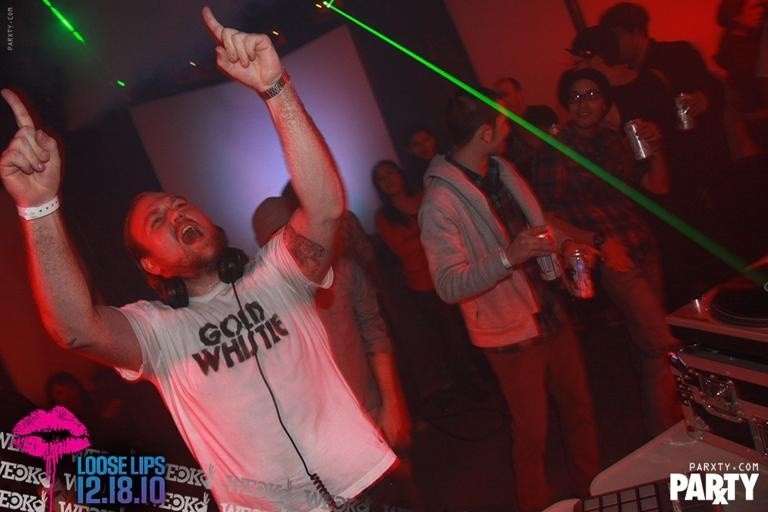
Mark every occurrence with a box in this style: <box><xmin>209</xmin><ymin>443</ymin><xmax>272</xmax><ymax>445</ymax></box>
<box><xmin>567</xmin><ymin>89</ymin><xmax>605</xmax><ymax>100</ymax></box>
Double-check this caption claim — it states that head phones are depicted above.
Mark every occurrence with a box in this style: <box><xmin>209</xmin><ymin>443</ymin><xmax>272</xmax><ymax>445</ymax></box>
<box><xmin>158</xmin><ymin>247</ymin><xmax>249</xmax><ymax>310</ymax></box>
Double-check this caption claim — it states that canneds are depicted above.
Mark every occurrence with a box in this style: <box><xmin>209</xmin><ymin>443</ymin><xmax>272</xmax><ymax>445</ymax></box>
<box><xmin>565</xmin><ymin>247</ymin><xmax>594</xmax><ymax>299</ymax></box>
<box><xmin>671</xmin><ymin>93</ymin><xmax>696</xmax><ymax>133</ymax></box>
<box><xmin>623</xmin><ymin>119</ymin><xmax>652</xmax><ymax>165</ymax></box>
<box><xmin>534</xmin><ymin>231</ymin><xmax>561</xmax><ymax>283</ymax></box>
